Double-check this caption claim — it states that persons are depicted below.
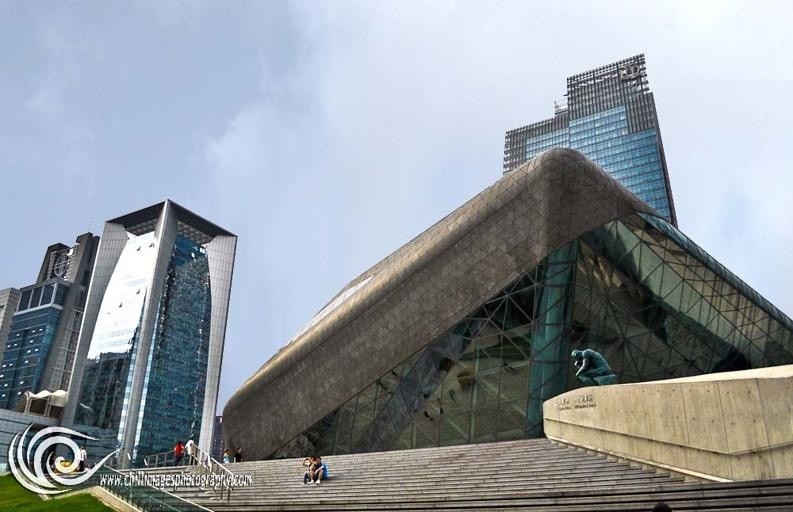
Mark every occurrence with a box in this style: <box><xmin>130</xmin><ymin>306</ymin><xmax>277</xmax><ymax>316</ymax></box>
<box><xmin>185</xmin><ymin>434</ymin><xmax>197</xmax><ymax>465</ymax></box>
<box><xmin>173</xmin><ymin>441</ymin><xmax>185</xmax><ymax>466</ymax></box>
<box><xmin>571</xmin><ymin>348</ymin><xmax>611</xmax><ymax>386</ymax></box>
<box><xmin>303</xmin><ymin>455</ymin><xmax>327</xmax><ymax>485</ymax></box>
<box><xmin>233</xmin><ymin>447</ymin><xmax>243</xmax><ymax>462</ymax></box>
<box><xmin>223</xmin><ymin>449</ymin><xmax>230</xmax><ymax>463</ymax></box>
<box><xmin>79</xmin><ymin>445</ymin><xmax>87</xmax><ymax>471</ymax></box>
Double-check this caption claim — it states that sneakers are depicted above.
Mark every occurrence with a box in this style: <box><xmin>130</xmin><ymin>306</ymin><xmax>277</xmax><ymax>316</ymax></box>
<box><xmin>310</xmin><ymin>479</ymin><xmax>320</xmax><ymax>485</ymax></box>
